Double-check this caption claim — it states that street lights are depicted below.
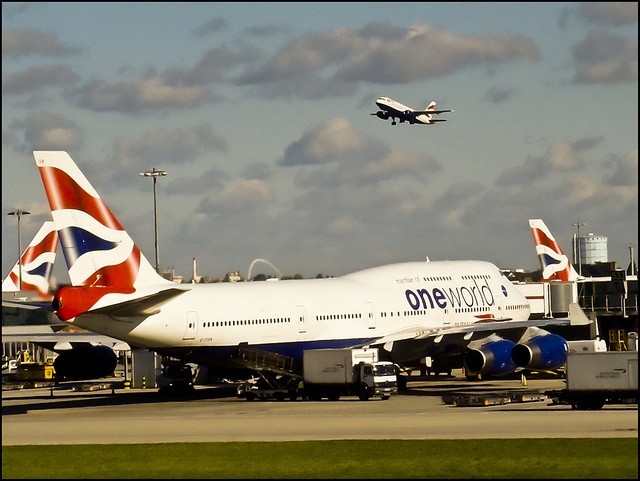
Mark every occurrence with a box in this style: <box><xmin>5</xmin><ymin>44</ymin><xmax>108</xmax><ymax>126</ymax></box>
<box><xmin>138</xmin><ymin>166</ymin><xmax>167</xmax><ymax>272</ymax></box>
<box><xmin>571</xmin><ymin>221</ymin><xmax>586</xmax><ymax>275</ymax></box>
<box><xmin>7</xmin><ymin>209</ymin><xmax>30</xmax><ymax>293</ymax></box>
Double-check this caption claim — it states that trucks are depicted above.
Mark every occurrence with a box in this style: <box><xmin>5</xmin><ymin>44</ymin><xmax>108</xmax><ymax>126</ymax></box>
<box><xmin>564</xmin><ymin>349</ymin><xmax>640</xmax><ymax>409</ymax></box>
<box><xmin>301</xmin><ymin>347</ymin><xmax>398</xmax><ymax>401</ymax></box>
<box><xmin>237</xmin><ymin>380</ymin><xmax>289</xmax><ymax>402</ymax></box>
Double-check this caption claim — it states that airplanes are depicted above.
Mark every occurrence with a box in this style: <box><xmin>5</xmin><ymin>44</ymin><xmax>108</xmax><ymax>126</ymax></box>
<box><xmin>32</xmin><ymin>147</ymin><xmax>595</xmax><ymax>384</ymax></box>
<box><xmin>370</xmin><ymin>95</ymin><xmax>453</xmax><ymax>125</ymax></box>
